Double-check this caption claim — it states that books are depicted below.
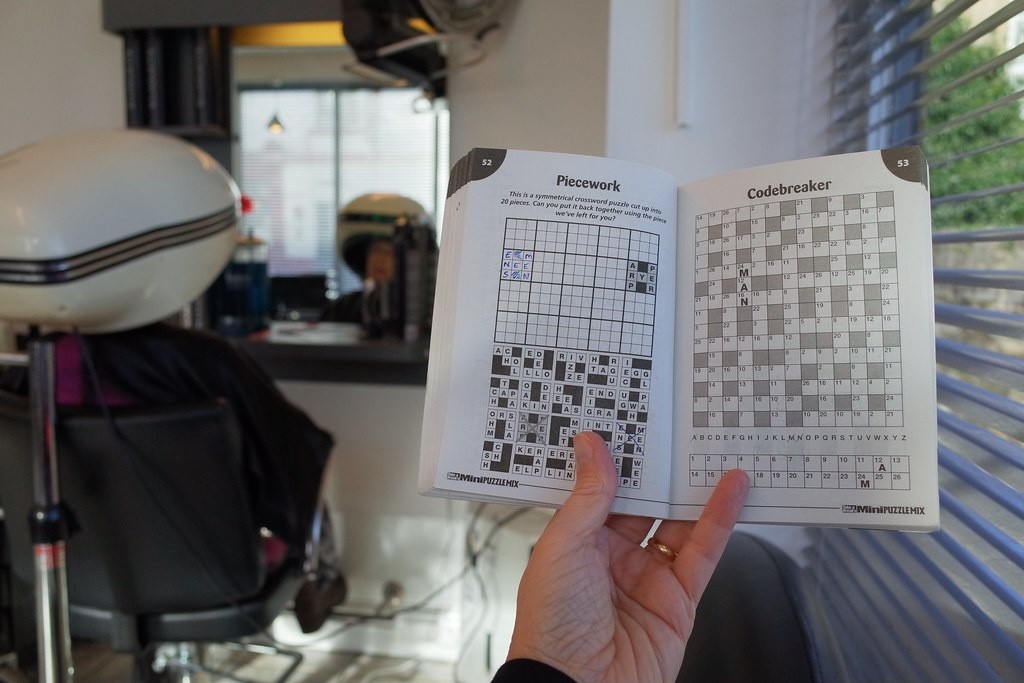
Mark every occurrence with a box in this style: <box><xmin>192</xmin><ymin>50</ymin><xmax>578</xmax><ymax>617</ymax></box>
<box><xmin>417</xmin><ymin>147</ymin><xmax>941</xmax><ymax>533</ymax></box>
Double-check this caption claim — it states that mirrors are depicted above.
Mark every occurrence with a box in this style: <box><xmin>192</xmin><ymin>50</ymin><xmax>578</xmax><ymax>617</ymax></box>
<box><xmin>235</xmin><ymin>82</ymin><xmax>452</xmax><ymax>322</ymax></box>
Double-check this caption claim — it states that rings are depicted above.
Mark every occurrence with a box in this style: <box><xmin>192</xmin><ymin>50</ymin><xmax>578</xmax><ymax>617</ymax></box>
<box><xmin>647</xmin><ymin>537</ymin><xmax>677</xmax><ymax>560</ymax></box>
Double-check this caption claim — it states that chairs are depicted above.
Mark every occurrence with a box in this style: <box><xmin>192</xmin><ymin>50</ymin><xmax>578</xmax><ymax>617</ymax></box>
<box><xmin>0</xmin><ymin>394</ymin><xmax>340</xmax><ymax>683</ymax></box>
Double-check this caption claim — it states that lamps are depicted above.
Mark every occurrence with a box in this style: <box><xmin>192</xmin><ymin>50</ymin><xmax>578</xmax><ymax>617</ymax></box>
<box><xmin>267</xmin><ymin>114</ymin><xmax>284</xmax><ymax>132</ymax></box>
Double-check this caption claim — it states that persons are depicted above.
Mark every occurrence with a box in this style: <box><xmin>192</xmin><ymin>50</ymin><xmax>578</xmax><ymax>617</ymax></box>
<box><xmin>320</xmin><ymin>239</ymin><xmax>397</xmax><ymax>321</ymax></box>
<box><xmin>490</xmin><ymin>432</ymin><xmax>815</xmax><ymax>683</ymax></box>
<box><xmin>54</xmin><ymin>331</ymin><xmax>336</xmax><ymax>565</ymax></box>
